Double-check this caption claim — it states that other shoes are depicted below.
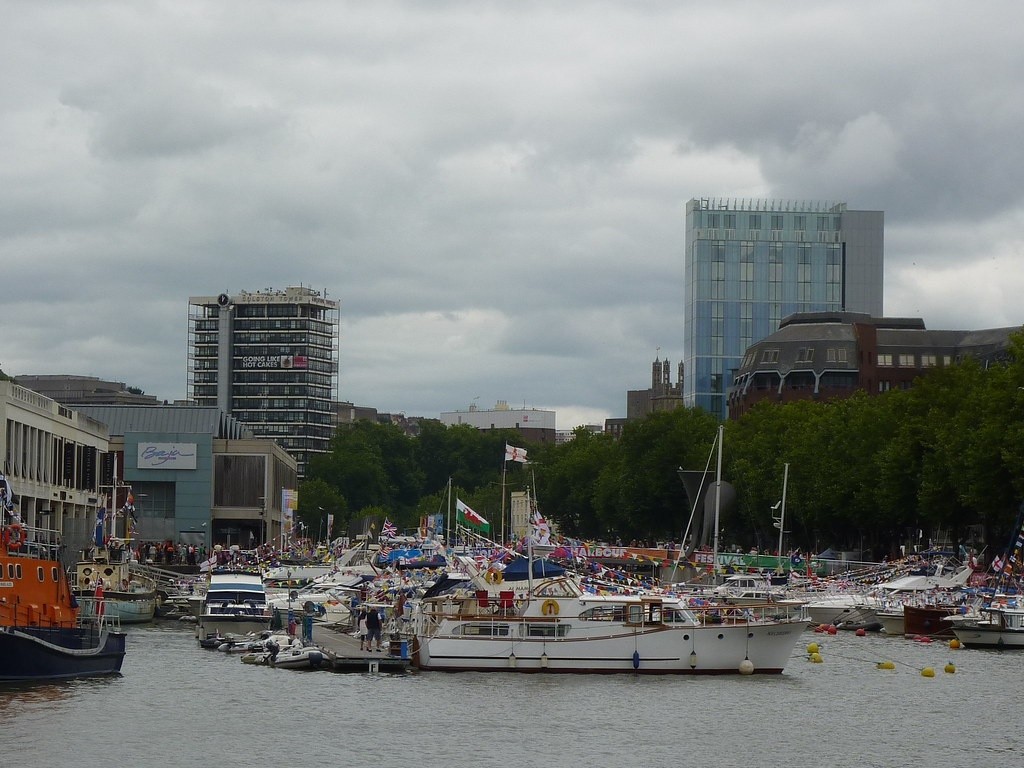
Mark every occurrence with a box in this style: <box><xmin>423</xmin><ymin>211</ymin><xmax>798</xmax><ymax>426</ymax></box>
<box><xmin>366</xmin><ymin>647</ymin><xmax>369</xmax><ymax>651</ymax></box>
<box><xmin>360</xmin><ymin>648</ymin><xmax>363</xmax><ymax>650</ymax></box>
<box><xmin>376</xmin><ymin>649</ymin><xmax>382</xmax><ymax>652</ymax></box>
<box><xmin>369</xmin><ymin>650</ymin><xmax>372</xmax><ymax>652</ymax></box>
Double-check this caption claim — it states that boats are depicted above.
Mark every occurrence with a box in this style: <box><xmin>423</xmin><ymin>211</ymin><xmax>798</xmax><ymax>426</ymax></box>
<box><xmin>1</xmin><ymin>449</ymin><xmax>157</xmax><ymax>681</ymax></box>
<box><xmin>196</xmin><ymin>424</ymin><xmax>1024</xmax><ymax>674</ymax></box>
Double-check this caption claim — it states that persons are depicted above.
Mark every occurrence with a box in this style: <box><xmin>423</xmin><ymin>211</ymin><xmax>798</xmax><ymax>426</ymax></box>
<box><xmin>349</xmin><ymin>593</ymin><xmax>383</xmax><ymax>652</ymax></box>
<box><xmin>117</xmin><ymin>579</ymin><xmax>129</xmax><ymax>592</ymax></box>
<box><xmin>546</xmin><ymin>534</ymin><xmax>684</xmax><ymax>550</ymax></box>
<box><xmin>132</xmin><ymin>541</ymin><xmax>276</xmax><ymax>565</ymax></box>
<box><xmin>388</xmin><ymin>542</ymin><xmax>423</xmax><ymax>550</ymax></box>
<box><xmin>175</xmin><ymin>577</ymin><xmax>197</xmax><ymax>596</ymax></box>
<box><xmin>883</xmin><ymin>556</ymin><xmax>887</xmax><ymax>564</ymax></box>
<box><xmin>283</xmin><ymin>539</ymin><xmax>342</xmax><ymax>561</ymax></box>
<box><xmin>684</xmin><ymin>544</ymin><xmax>819</xmax><ymax>560</ymax></box>
<box><xmin>933</xmin><ymin>584</ymin><xmax>943</xmax><ymax>592</ymax></box>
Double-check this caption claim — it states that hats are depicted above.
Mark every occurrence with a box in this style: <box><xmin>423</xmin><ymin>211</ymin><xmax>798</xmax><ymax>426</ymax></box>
<box><xmin>370</xmin><ymin>607</ymin><xmax>377</xmax><ymax>612</ymax></box>
<box><xmin>361</xmin><ymin>606</ymin><xmax>367</xmax><ymax>612</ymax></box>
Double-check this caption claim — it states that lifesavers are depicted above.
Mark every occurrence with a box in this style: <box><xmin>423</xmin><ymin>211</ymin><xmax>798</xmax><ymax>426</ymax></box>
<box><xmin>4</xmin><ymin>524</ymin><xmax>25</xmax><ymax>549</ymax></box>
<box><xmin>542</xmin><ymin>600</ymin><xmax>559</xmax><ymax>615</ymax></box>
<box><xmin>486</xmin><ymin>568</ymin><xmax>503</xmax><ymax>584</ymax></box>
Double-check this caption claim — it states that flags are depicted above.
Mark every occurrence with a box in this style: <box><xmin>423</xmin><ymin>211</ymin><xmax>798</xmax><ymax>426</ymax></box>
<box><xmin>382</xmin><ymin>516</ymin><xmax>397</xmax><ymax>539</ymax></box>
<box><xmin>393</xmin><ymin>590</ymin><xmax>407</xmax><ymax>618</ymax></box>
<box><xmin>789</xmin><ymin>566</ymin><xmax>800</xmax><ymax>582</ymax></box>
<box><xmin>505</xmin><ymin>444</ymin><xmax>528</xmax><ymax>463</ymax></box>
<box><xmin>456</xmin><ymin>497</ymin><xmax>490</xmax><ymax>534</ymax></box>
<box><xmin>378</xmin><ymin>543</ymin><xmax>392</xmax><ymax>558</ymax></box>
<box><xmin>528</xmin><ymin>508</ymin><xmax>551</xmax><ymax>547</ymax></box>
<box><xmin>991</xmin><ymin>525</ymin><xmax>1024</xmax><ymax>580</ymax></box>
<box><xmin>790</xmin><ymin>547</ymin><xmax>806</xmax><ymax>565</ymax></box>
